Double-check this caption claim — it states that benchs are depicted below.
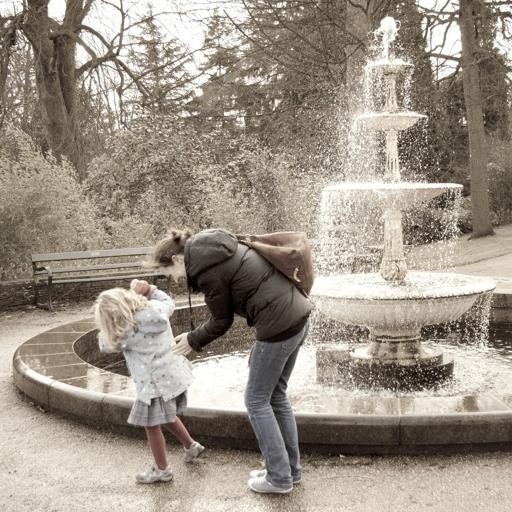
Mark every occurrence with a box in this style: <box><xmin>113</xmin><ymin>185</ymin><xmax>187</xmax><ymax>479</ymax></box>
<box><xmin>31</xmin><ymin>246</ymin><xmax>171</xmax><ymax>312</ymax></box>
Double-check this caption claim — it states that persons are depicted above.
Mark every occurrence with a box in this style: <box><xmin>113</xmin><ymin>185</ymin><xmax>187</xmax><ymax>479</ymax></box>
<box><xmin>90</xmin><ymin>277</ymin><xmax>206</xmax><ymax>486</ymax></box>
<box><xmin>137</xmin><ymin>223</ymin><xmax>315</xmax><ymax>497</ymax></box>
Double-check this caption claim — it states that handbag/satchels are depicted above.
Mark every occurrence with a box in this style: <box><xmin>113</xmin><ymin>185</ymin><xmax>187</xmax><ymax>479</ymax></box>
<box><xmin>233</xmin><ymin>230</ymin><xmax>315</xmax><ymax>295</ymax></box>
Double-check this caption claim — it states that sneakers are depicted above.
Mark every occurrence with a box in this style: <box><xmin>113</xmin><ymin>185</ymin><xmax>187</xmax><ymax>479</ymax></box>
<box><xmin>247</xmin><ymin>478</ymin><xmax>295</xmax><ymax>496</ymax></box>
<box><xmin>249</xmin><ymin>467</ymin><xmax>302</xmax><ymax>484</ymax></box>
<box><xmin>183</xmin><ymin>441</ymin><xmax>206</xmax><ymax>465</ymax></box>
<box><xmin>134</xmin><ymin>465</ymin><xmax>174</xmax><ymax>484</ymax></box>
